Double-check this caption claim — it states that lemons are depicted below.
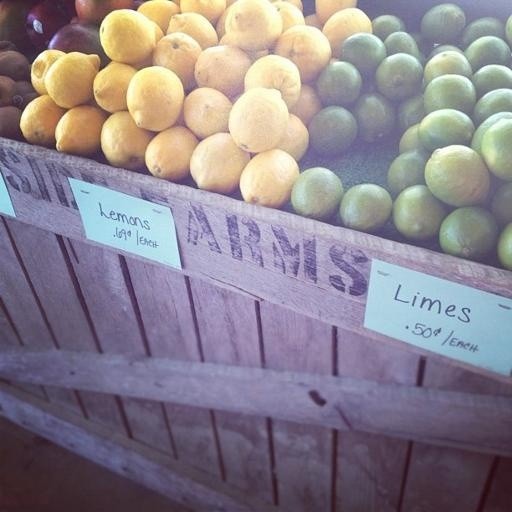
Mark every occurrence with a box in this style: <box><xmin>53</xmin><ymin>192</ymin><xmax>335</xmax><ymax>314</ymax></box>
<box><xmin>20</xmin><ymin>0</ymin><xmax>512</xmax><ymax>272</ymax></box>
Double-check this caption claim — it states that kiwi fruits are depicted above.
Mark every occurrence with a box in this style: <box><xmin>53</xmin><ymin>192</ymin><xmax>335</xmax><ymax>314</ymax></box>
<box><xmin>0</xmin><ymin>1</ymin><xmax>39</xmax><ymax>139</ymax></box>
